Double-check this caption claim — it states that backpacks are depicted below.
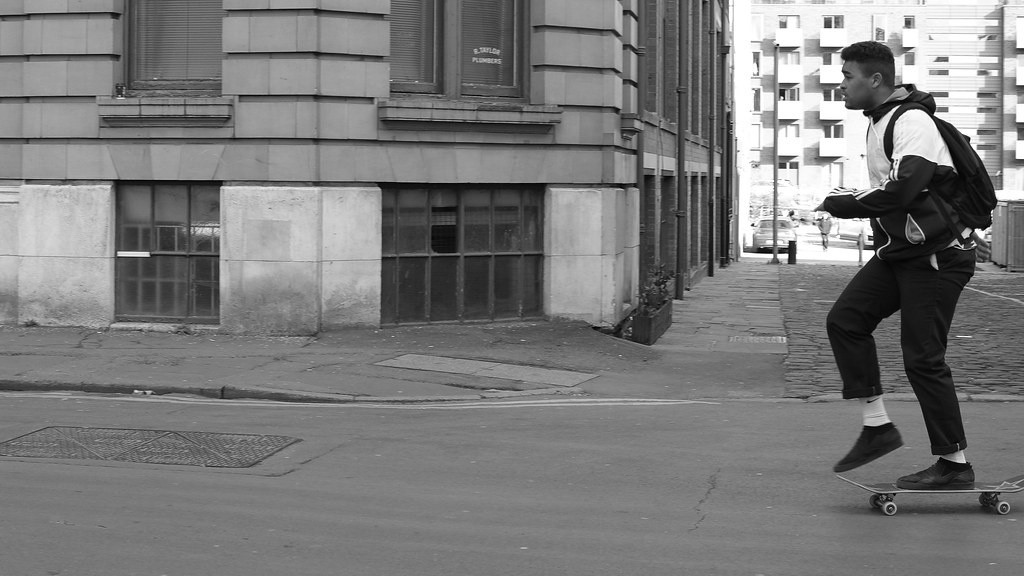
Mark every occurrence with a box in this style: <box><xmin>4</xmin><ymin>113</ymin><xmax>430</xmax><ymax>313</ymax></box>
<box><xmin>884</xmin><ymin>101</ymin><xmax>998</xmax><ymax>230</ymax></box>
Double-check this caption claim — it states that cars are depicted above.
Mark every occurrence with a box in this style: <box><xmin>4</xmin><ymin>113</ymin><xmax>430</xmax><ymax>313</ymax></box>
<box><xmin>750</xmin><ymin>216</ymin><xmax>800</xmax><ymax>253</ymax></box>
<box><xmin>760</xmin><ymin>207</ymin><xmax>874</xmax><ymax>250</ymax></box>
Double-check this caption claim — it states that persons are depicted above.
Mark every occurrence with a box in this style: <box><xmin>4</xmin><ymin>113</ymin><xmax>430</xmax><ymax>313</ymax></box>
<box><xmin>818</xmin><ymin>214</ymin><xmax>831</xmax><ymax>249</ymax></box>
<box><xmin>787</xmin><ymin>211</ymin><xmax>795</xmax><ymax>221</ymax></box>
<box><xmin>812</xmin><ymin>42</ymin><xmax>976</xmax><ymax>491</ymax></box>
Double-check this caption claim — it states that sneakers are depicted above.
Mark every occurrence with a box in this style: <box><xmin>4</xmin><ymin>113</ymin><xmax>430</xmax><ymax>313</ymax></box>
<box><xmin>896</xmin><ymin>458</ymin><xmax>975</xmax><ymax>490</ymax></box>
<box><xmin>834</xmin><ymin>428</ymin><xmax>903</xmax><ymax>472</ymax></box>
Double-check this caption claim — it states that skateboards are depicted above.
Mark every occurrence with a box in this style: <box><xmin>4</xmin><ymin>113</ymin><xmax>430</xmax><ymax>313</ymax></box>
<box><xmin>835</xmin><ymin>473</ymin><xmax>1024</xmax><ymax>516</ymax></box>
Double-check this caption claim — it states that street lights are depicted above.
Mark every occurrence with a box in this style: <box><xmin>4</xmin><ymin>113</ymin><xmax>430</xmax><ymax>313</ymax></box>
<box><xmin>770</xmin><ymin>43</ymin><xmax>801</xmax><ymax>264</ymax></box>
